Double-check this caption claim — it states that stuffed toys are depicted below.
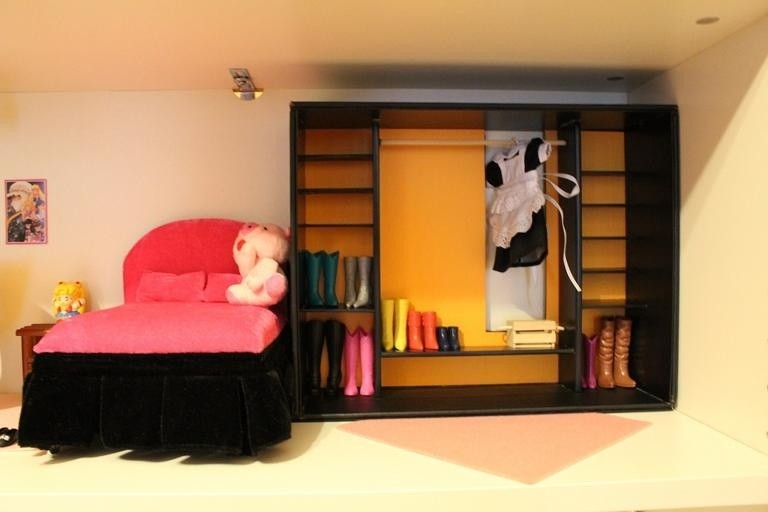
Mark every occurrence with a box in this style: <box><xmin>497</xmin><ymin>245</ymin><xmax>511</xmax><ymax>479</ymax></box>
<box><xmin>49</xmin><ymin>280</ymin><xmax>87</xmax><ymax>322</ymax></box>
<box><xmin>224</xmin><ymin>221</ymin><xmax>291</xmax><ymax>306</ymax></box>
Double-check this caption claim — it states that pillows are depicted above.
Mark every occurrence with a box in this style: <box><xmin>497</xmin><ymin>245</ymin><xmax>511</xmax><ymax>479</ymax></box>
<box><xmin>134</xmin><ymin>270</ymin><xmax>242</xmax><ymax>303</ymax></box>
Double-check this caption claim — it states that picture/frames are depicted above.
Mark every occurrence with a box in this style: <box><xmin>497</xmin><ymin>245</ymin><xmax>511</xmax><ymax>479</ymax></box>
<box><xmin>4</xmin><ymin>179</ymin><xmax>48</xmax><ymax>245</ymax></box>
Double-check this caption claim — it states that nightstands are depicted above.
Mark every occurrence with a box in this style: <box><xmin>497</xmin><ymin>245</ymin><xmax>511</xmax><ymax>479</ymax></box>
<box><xmin>15</xmin><ymin>323</ymin><xmax>54</xmax><ymax>382</ymax></box>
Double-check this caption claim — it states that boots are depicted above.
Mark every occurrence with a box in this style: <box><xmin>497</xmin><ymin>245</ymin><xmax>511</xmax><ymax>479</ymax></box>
<box><xmin>581</xmin><ymin>317</ymin><xmax>638</xmax><ymax>389</ymax></box>
<box><xmin>381</xmin><ymin>299</ymin><xmax>461</xmax><ymax>352</ymax></box>
<box><xmin>302</xmin><ymin>249</ymin><xmax>372</xmax><ymax>308</ymax></box>
<box><xmin>305</xmin><ymin>319</ymin><xmax>375</xmax><ymax>397</ymax></box>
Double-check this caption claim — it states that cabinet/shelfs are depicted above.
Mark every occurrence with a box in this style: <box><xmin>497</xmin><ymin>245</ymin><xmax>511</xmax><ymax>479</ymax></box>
<box><xmin>380</xmin><ymin>105</ymin><xmax>573</xmax><ymax>354</ymax></box>
<box><xmin>379</xmin><ymin>353</ymin><xmax>576</xmax><ymax>418</ymax></box>
<box><xmin>291</xmin><ymin>102</ymin><xmax>379</xmax><ymax>399</ymax></box>
<box><xmin>578</xmin><ymin>109</ymin><xmax>651</xmax><ymax>392</ymax></box>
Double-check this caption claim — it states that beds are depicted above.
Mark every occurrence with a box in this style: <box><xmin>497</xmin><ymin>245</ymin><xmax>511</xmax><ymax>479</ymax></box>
<box><xmin>18</xmin><ymin>218</ymin><xmax>291</xmax><ymax>462</ymax></box>
